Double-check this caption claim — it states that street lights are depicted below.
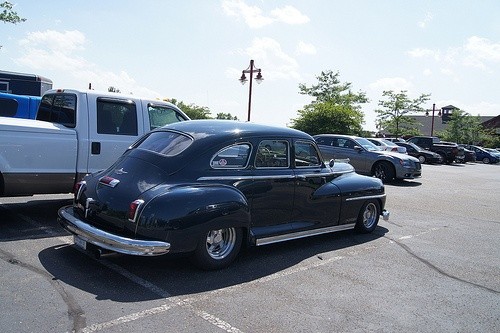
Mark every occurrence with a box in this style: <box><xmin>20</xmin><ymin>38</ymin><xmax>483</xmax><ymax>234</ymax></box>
<box><xmin>425</xmin><ymin>104</ymin><xmax>442</xmax><ymax>137</ymax></box>
<box><xmin>239</xmin><ymin>59</ymin><xmax>264</xmax><ymax>121</ymax></box>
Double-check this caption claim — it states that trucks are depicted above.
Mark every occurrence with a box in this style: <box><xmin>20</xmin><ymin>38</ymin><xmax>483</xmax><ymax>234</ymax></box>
<box><xmin>0</xmin><ymin>87</ymin><xmax>193</xmax><ymax>208</ymax></box>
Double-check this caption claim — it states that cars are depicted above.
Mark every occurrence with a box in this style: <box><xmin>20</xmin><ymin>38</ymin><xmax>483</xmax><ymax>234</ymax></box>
<box><xmin>339</xmin><ymin>137</ymin><xmax>409</xmax><ymax>156</ymax></box>
<box><xmin>259</xmin><ymin>139</ymin><xmax>287</xmax><ymax>152</ymax></box>
<box><xmin>384</xmin><ymin>136</ymin><xmax>500</xmax><ymax>166</ymax></box>
<box><xmin>57</xmin><ymin>118</ymin><xmax>390</xmax><ymax>271</ymax></box>
<box><xmin>293</xmin><ymin>134</ymin><xmax>422</xmax><ymax>185</ymax></box>
